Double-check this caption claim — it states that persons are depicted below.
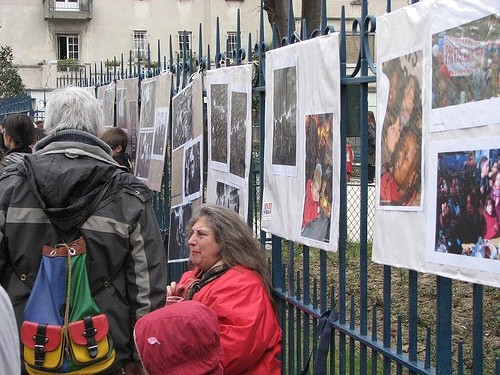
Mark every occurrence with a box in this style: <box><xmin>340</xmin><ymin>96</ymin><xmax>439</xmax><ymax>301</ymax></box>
<box><xmin>132</xmin><ymin>203</ymin><xmax>282</xmax><ymax>375</ymax></box>
<box><xmin>380</xmin><ymin>51</ymin><xmax>422</xmax><ymax>206</ymax></box>
<box><xmin>0</xmin><ymin>96</ymin><xmax>168</xmax><ymax>374</ymax></box>
<box><xmin>0</xmin><ymin>111</ymin><xmax>36</xmax><ymax>170</ymax></box>
<box><xmin>100</xmin><ymin>128</ymin><xmax>133</xmax><ymax>176</ymax></box>
<box><xmin>135</xmin><ymin>133</ymin><xmax>153</xmax><ymax>179</ymax></box>
<box><xmin>185</xmin><ymin>141</ymin><xmax>204</xmax><ymax>198</ymax></box>
<box><xmin>435</xmin><ymin>148</ymin><xmax>500</xmax><ymax>260</ymax></box>
<box><xmin>367</xmin><ymin>111</ymin><xmax>376</xmax><ymax>184</ymax></box>
<box><xmin>345</xmin><ymin>140</ymin><xmax>354</xmax><ymax>183</ymax></box>
<box><xmin>300</xmin><ymin>161</ymin><xmax>333</xmax><ymax>243</ymax></box>
<box><xmin>0</xmin><ymin>286</ymin><xmax>21</xmax><ymax>375</ymax></box>
<box><xmin>432</xmin><ymin>14</ymin><xmax>500</xmax><ymax>109</ymax></box>
<box><xmin>0</xmin><ymin>118</ymin><xmax>48</xmax><ymax>162</ymax></box>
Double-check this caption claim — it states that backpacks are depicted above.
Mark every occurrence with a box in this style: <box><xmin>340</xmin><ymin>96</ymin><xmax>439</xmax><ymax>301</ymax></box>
<box><xmin>8</xmin><ymin>163</ymin><xmax>129</xmax><ymax>375</ymax></box>
